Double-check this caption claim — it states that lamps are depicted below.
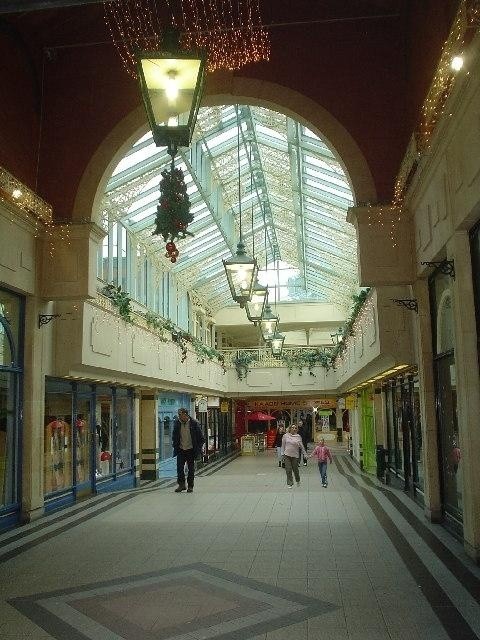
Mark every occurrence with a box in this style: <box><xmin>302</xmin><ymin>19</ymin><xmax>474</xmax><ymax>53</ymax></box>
<box><xmin>137</xmin><ymin>2</ymin><xmax>207</xmax><ymax>157</ymax></box>
<box><xmin>221</xmin><ymin>104</ymin><xmax>285</xmax><ymax>360</ymax></box>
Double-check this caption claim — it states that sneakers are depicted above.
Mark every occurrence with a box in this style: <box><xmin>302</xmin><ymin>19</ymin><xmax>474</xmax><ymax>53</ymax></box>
<box><xmin>187</xmin><ymin>487</ymin><xmax>193</xmax><ymax>493</ymax></box>
<box><xmin>175</xmin><ymin>486</ymin><xmax>187</xmax><ymax>492</ymax></box>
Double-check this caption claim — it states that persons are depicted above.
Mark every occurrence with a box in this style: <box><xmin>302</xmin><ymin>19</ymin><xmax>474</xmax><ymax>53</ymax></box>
<box><xmin>281</xmin><ymin>424</ymin><xmax>309</xmax><ymax>489</ymax></box>
<box><xmin>272</xmin><ymin>424</ymin><xmax>286</xmax><ymax>468</ymax></box>
<box><xmin>309</xmin><ymin>437</ymin><xmax>332</xmax><ymax>488</ymax></box>
<box><xmin>297</xmin><ymin>420</ymin><xmax>309</xmax><ymax>467</ymax></box>
<box><xmin>172</xmin><ymin>409</ymin><xmax>205</xmax><ymax>493</ymax></box>
<box><xmin>410</xmin><ymin>414</ymin><xmax>422</xmax><ymax>463</ymax></box>
<box><xmin>452</xmin><ymin>441</ymin><xmax>462</xmax><ymax>473</ymax></box>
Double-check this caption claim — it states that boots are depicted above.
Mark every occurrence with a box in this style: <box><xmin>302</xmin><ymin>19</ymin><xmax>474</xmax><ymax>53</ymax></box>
<box><xmin>279</xmin><ymin>461</ymin><xmax>281</xmax><ymax>467</ymax></box>
<box><xmin>282</xmin><ymin>463</ymin><xmax>285</xmax><ymax>468</ymax></box>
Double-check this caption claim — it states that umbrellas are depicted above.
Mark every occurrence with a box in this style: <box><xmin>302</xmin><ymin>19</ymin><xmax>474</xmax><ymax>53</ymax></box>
<box><xmin>243</xmin><ymin>412</ymin><xmax>276</xmax><ymax>421</ymax></box>
